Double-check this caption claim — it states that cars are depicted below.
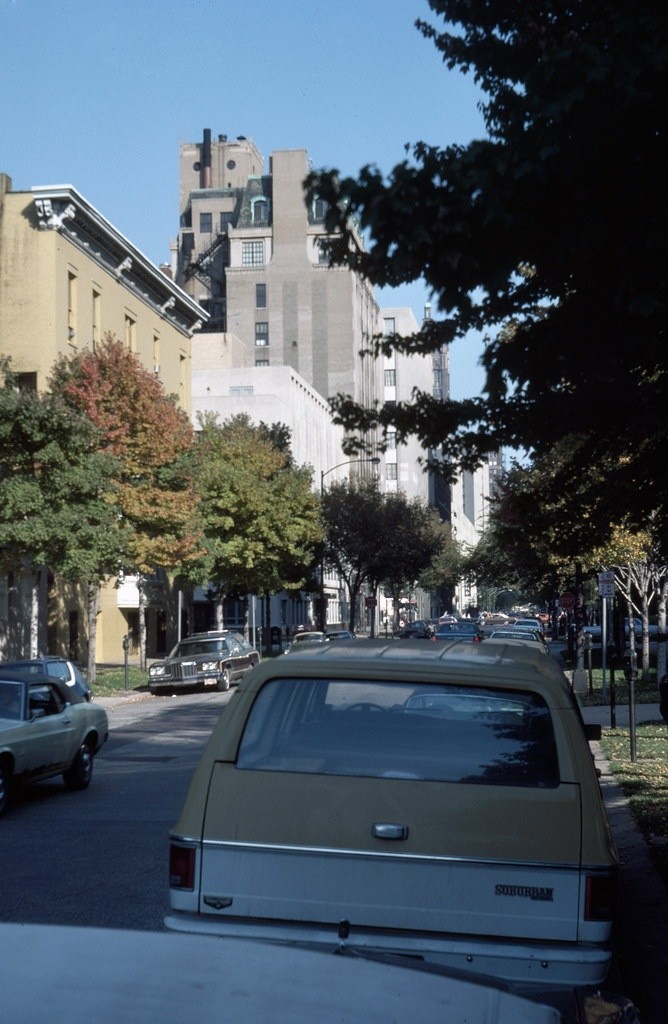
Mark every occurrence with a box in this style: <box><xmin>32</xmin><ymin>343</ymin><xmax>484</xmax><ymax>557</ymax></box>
<box><xmin>399</xmin><ymin>611</ymin><xmax>550</xmax><ymax>655</ymax></box>
<box><xmin>284</xmin><ymin>630</ymin><xmax>356</xmax><ymax>654</ymax></box>
<box><xmin>581</xmin><ymin>618</ymin><xmax>668</xmax><ymax>642</ymax></box>
<box><xmin>1</xmin><ymin>671</ymin><xmax>109</xmax><ymax>809</ymax></box>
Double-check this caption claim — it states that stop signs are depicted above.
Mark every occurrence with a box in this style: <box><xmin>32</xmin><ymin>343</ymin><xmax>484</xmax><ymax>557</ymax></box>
<box><xmin>559</xmin><ymin>592</ymin><xmax>575</xmax><ymax>609</ymax></box>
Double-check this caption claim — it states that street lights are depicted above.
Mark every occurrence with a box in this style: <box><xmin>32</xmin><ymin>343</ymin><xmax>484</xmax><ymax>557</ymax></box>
<box><xmin>319</xmin><ymin>458</ymin><xmax>380</xmax><ymax>630</ymax></box>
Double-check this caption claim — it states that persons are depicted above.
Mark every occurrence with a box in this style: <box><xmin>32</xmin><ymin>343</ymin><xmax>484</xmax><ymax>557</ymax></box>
<box><xmin>399</xmin><ymin>618</ymin><xmax>404</xmax><ymax>630</ymax></box>
<box><xmin>477</xmin><ymin>610</ymin><xmax>494</xmax><ymax>625</ymax></box>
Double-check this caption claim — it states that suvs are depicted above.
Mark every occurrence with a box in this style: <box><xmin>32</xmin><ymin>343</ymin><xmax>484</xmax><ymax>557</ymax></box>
<box><xmin>0</xmin><ymin>656</ymin><xmax>94</xmax><ymax>704</ymax></box>
<box><xmin>148</xmin><ymin>630</ymin><xmax>261</xmax><ymax>692</ymax></box>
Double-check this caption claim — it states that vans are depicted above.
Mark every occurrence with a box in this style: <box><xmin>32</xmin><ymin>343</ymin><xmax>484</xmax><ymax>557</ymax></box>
<box><xmin>164</xmin><ymin>639</ymin><xmax>622</xmax><ymax>1001</ymax></box>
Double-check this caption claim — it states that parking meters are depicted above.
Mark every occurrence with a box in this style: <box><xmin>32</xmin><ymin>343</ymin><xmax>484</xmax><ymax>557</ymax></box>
<box><xmin>624</xmin><ymin>649</ymin><xmax>639</xmax><ymax>762</ymax></box>
<box><xmin>583</xmin><ymin>633</ymin><xmax>595</xmax><ymax>695</ymax></box>
<box><xmin>123</xmin><ymin>635</ymin><xmax>130</xmax><ymax>688</ymax></box>
<box><xmin>606</xmin><ymin>640</ymin><xmax>617</xmax><ymax>728</ymax></box>
